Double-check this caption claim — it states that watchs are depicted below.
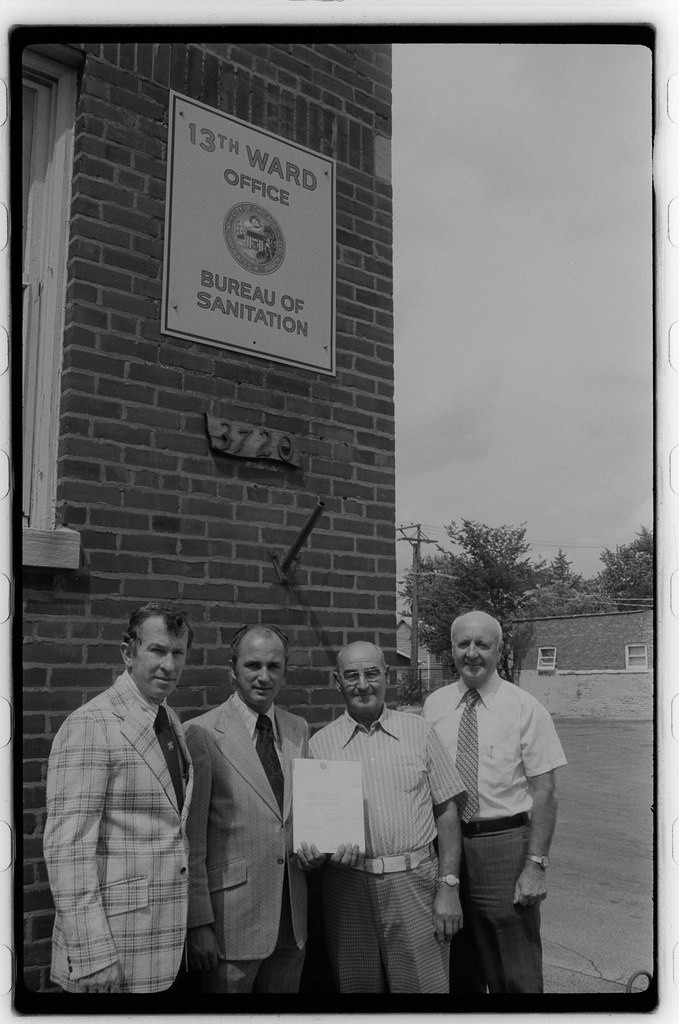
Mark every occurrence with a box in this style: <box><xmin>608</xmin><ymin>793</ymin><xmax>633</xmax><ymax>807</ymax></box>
<box><xmin>525</xmin><ymin>854</ymin><xmax>549</xmax><ymax>869</ymax></box>
<box><xmin>439</xmin><ymin>874</ymin><xmax>460</xmax><ymax>886</ymax></box>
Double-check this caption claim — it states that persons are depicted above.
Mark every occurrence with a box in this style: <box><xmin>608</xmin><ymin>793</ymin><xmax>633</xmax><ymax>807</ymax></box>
<box><xmin>181</xmin><ymin>623</ymin><xmax>326</xmax><ymax>994</ymax></box>
<box><xmin>43</xmin><ymin>600</ymin><xmax>195</xmax><ymax>995</ymax></box>
<box><xmin>420</xmin><ymin>611</ymin><xmax>567</xmax><ymax>994</ymax></box>
<box><xmin>307</xmin><ymin>641</ymin><xmax>466</xmax><ymax>994</ymax></box>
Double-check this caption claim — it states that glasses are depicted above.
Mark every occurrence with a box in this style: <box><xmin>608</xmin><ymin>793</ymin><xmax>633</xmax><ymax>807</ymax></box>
<box><xmin>339</xmin><ymin>666</ymin><xmax>385</xmax><ymax>685</ymax></box>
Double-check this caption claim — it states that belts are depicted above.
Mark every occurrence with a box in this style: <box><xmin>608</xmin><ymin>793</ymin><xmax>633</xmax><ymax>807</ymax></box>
<box><xmin>456</xmin><ymin>812</ymin><xmax>530</xmax><ymax>836</ymax></box>
<box><xmin>350</xmin><ymin>842</ymin><xmax>435</xmax><ymax>876</ymax></box>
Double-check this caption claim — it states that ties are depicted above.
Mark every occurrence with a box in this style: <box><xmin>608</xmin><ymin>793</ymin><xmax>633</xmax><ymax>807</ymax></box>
<box><xmin>255</xmin><ymin>715</ymin><xmax>284</xmax><ymax>818</ymax></box>
<box><xmin>153</xmin><ymin>706</ymin><xmax>185</xmax><ymax>816</ymax></box>
<box><xmin>454</xmin><ymin>688</ymin><xmax>481</xmax><ymax>824</ymax></box>
